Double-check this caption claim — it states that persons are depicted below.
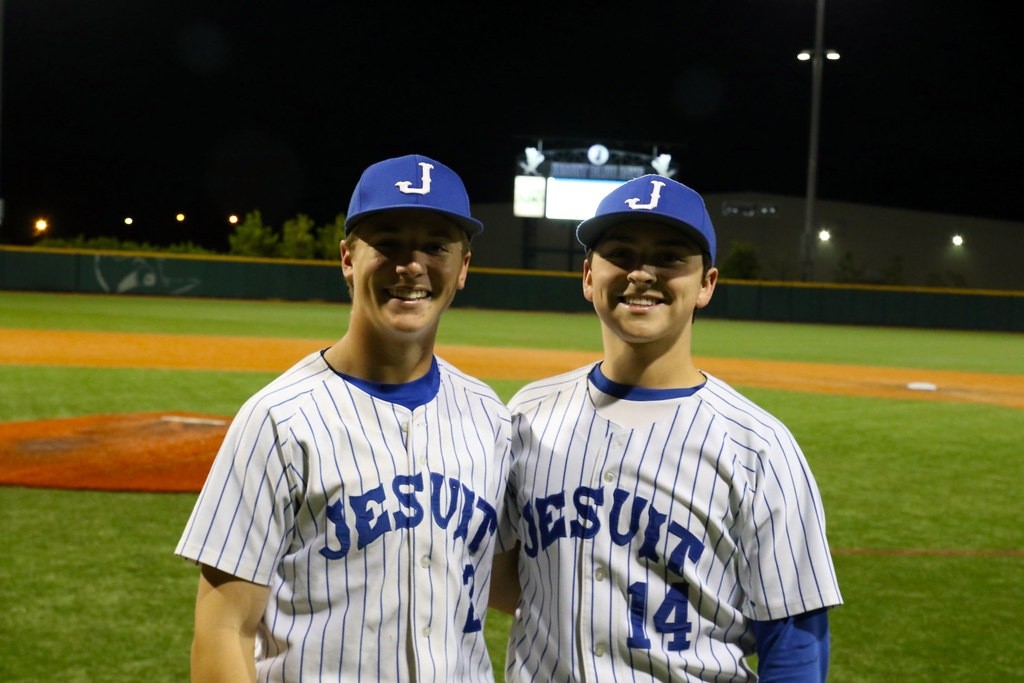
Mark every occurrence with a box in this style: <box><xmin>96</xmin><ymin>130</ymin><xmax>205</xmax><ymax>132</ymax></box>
<box><xmin>173</xmin><ymin>152</ymin><xmax>511</xmax><ymax>683</ymax></box>
<box><xmin>488</xmin><ymin>176</ymin><xmax>842</xmax><ymax>682</ymax></box>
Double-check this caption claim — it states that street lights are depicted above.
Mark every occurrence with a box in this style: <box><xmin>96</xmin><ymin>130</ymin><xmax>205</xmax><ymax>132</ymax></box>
<box><xmin>797</xmin><ymin>44</ymin><xmax>844</xmax><ymax>287</ymax></box>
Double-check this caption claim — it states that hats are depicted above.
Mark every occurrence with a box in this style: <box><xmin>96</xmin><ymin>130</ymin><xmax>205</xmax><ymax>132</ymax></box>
<box><xmin>345</xmin><ymin>154</ymin><xmax>484</xmax><ymax>235</ymax></box>
<box><xmin>575</xmin><ymin>175</ymin><xmax>717</xmax><ymax>267</ymax></box>
